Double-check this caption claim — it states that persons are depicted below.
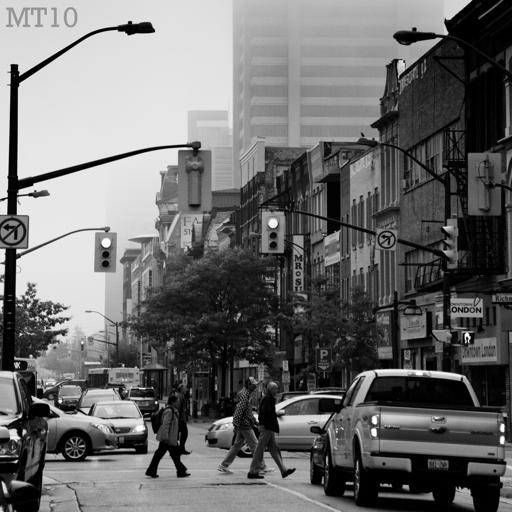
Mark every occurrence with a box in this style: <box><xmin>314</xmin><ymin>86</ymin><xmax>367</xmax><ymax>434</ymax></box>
<box><xmin>149</xmin><ymin>377</ymin><xmax>244</xmax><ymax>422</ymax></box>
<box><xmin>217</xmin><ymin>375</ymin><xmax>277</xmax><ymax>474</ymax></box>
<box><xmin>171</xmin><ymin>383</ymin><xmax>190</xmax><ymax>455</ymax></box>
<box><xmin>144</xmin><ymin>394</ymin><xmax>191</xmax><ymax>478</ymax></box>
<box><xmin>247</xmin><ymin>382</ymin><xmax>297</xmax><ymax>479</ymax></box>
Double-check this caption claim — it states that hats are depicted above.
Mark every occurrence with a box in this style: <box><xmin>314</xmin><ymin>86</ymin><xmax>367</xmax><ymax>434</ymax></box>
<box><xmin>248</xmin><ymin>376</ymin><xmax>259</xmax><ymax>385</ymax></box>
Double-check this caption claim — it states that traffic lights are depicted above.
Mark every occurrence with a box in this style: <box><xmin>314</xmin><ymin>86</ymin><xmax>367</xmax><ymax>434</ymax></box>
<box><xmin>95</xmin><ymin>232</ymin><xmax>117</xmax><ymax>274</ymax></box>
<box><xmin>461</xmin><ymin>331</ymin><xmax>476</xmax><ymax>346</ymax></box>
<box><xmin>442</xmin><ymin>218</ymin><xmax>459</xmax><ymax>270</ymax></box>
<box><xmin>429</xmin><ymin>329</ymin><xmax>451</xmax><ymax>346</ymax></box>
<box><xmin>262</xmin><ymin>213</ymin><xmax>285</xmax><ymax>253</ymax></box>
<box><xmin>80</xmin><ymin>338</ymin><xmax>84</xmax><ymax>351</ymax></box>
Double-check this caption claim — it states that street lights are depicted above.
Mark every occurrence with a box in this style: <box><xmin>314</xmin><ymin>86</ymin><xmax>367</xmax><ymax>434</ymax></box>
<box><xmin>6</xmin><ymin>19</ymin><xmax>156</xmax><ymax>507</ymax></box>
<box><xmin>358</xmin><ymin>136</ymin><xmax>452</xmax><ymax>371</ymax></box>
<box><xmin>373</xmin><ymin>291</ymin><xmax>423</xmax><ymax>367</ymax></box>
<box><xmin>85</xmin><ymin>310</ymin><xmax>119</xmax><ymax>362</ymax></box>
<box><xmin>0</xmin><ymin>190</ymin><xmax>50</xmax><ymax>201</ymax></box>
<box><xmin>392</xmin><ymin>27</ymin><xmax>512</xmax><ymax>80</ymax></box>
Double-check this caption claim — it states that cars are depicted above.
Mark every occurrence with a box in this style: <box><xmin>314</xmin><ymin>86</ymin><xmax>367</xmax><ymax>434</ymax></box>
<box><xmin>0</xmin><ymin>370</ymin><xmax>49</xmax><ymax>512</ymax></box>
<box><xmin>43</xmin><ymin>367</ymin><xmax>160</xmax><ymax>413</ymax></box>
<box><xmin>308</xmin><ymin>410</ymin><xmax>404</xmax><ymax>490</ymax></box>
<box><xmin>30</xmin><ymin>395</ymin><xmax>118</xmax><ymax>462</ymax></box>
<box><xmin>87</xmin><ymin>402</ymin><xmax>148</xmax><ymax>455</ymax></box>
<box><xmin>277</xmin><ymin>387</ymin><xmax>347</xmax><ymax>411</ymax></box>
<box><xmin>0</xmin><ymin>480</ymin><xmax>39</xmax><ymax>512</ymax></box>
<box><xmin>204</xmin><ymin>396</ymin><xmax>344</xmax><ymax>460</ymax></box>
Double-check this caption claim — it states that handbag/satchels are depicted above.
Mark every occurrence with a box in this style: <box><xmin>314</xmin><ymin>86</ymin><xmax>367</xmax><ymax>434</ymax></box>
<box><xmin>151</xmin><ymin>407</ymin><xmax>164</xmax><ymax>433</ymax></box>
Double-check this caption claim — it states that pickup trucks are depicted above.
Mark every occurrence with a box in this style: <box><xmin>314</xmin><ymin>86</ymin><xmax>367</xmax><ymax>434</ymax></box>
<box><xmin>321</xmin><ymin>368</ymin><xmax>508</xmax><ymax>512</ymax></box>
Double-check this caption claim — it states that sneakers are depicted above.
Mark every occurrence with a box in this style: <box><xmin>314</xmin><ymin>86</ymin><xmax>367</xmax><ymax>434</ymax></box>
<box><xmin>145</xmin><ymin>471</ymin><xmax>159</xmax><ymax>478</ymax></box>
<box><xmin>216</xmin><ymin>463</ymin><xmax>235</xmax><ymax>474</ymax></box>
<box><xmin>180</xmin><ymin>450</ymin><xmax>191</xmax><ymax>454</ymax></box>
<box><xmin>259</xmin><ymin>467</ymin><xmax>273</xmax><ymax>475</ymax></box>
<box><xmin>247</xmin><ymin>472</ymin><xmax>264</xmax><ymax>479</ymax></box>
<box><xmin>177</xmin><ymin>472</ymin><xmax>191</xmax><ymax>477</ymax></box>
<box><xmin>282</xmin><ymin>467</ymin><xmax>296</xmax><ymax>478</ymax></box>
